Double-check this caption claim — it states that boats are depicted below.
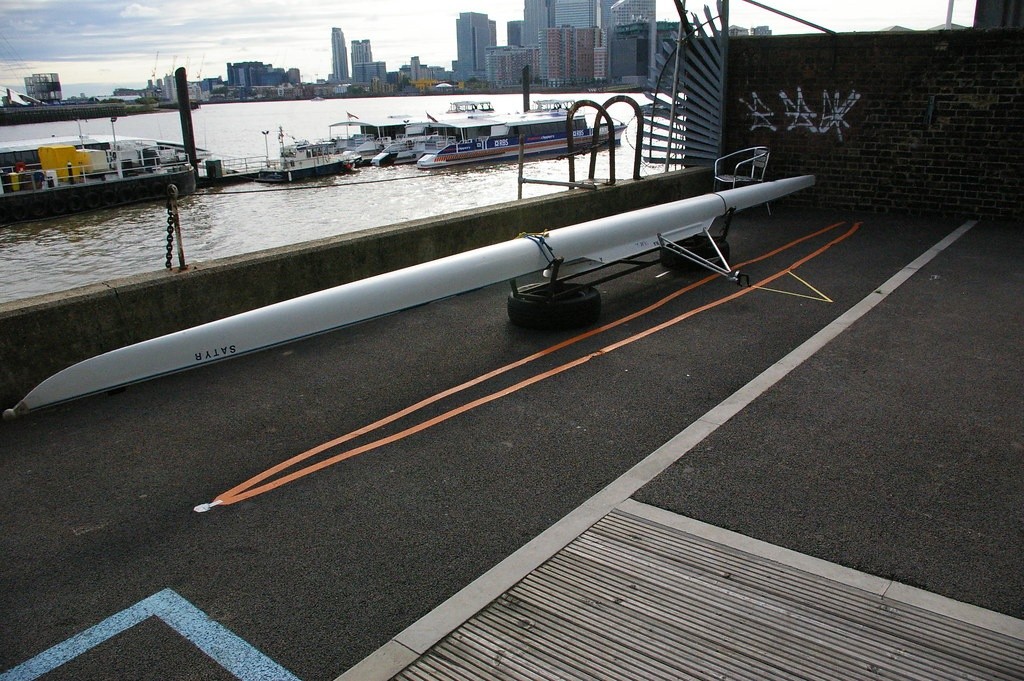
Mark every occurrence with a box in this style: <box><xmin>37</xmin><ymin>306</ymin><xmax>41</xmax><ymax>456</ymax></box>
<box><xmin>635</xmin><ymin>102</ymin><xmax>686</xmax><ymax>120</ymax></box>
<box><xmin>530</xmin><ymin>100</ymin><xmax>581</xmax><ymax>114</ymax></box>
<box><xmin>318</xmin><ymin>109</ymin><xmax>628</xmax><ymax>170</ymax></box>
<box><xmin>0</xmin><ymin>134</ymin><xmax>214</xmax><ymax>227</ymax></box>
<box><xmin>0</xmin><ymin>97</ymin><xmax>128</xmax><ymax>125</ymax></box>
<box><xmin>444</xmin><ymin>101</ymin><xmax>495</xmax><ymax>116</ymax></box>
<box><xmin>254</xmin><ymin>131</ymin><xmax>360</xmax><ymax>183</ymax></box>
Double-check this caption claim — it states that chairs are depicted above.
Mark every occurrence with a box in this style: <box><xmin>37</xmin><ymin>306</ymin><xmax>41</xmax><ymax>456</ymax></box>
<box><xmin>713</xmin><ymin>145</ymin><xmax>772</xmax><ymax>216</ymax></box>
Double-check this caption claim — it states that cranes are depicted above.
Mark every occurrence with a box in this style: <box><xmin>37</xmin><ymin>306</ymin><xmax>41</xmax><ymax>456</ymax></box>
<box><xmin>151</xmin><ymin>50</ymin><xmax>161</xmax><ymax>86</ymax></box>
<box><xmin>165</xmin><ymin>53</ymin><xmax>207</xmax><ymax>80</ymax></box>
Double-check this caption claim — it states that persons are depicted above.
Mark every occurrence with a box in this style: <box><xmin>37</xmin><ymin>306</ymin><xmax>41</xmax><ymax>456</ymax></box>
<box><xmin>474</xmin><ymin>105</ymin><xmax>477</xmax><ymax>109</ymax></box>
<box><xmin>281</xmin><ymin>152</ymin><xmax>284</xmax><ymax>156</ymax></box>
<box><xmin>380</xmin><ymin>142</ymin><xmax>385</xmax><ymax>149</ymax></box>
<box><xmin>288</xmin><ymin>149</ymin><xmax>294</xmax><ymax>166</ymax></box>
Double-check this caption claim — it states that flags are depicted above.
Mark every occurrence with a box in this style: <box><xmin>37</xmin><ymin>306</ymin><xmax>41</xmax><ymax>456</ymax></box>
<box><xmin>347</xmin><ymin>112</ymin><xmax>359</xmax><ymax>119</ymax></box>
